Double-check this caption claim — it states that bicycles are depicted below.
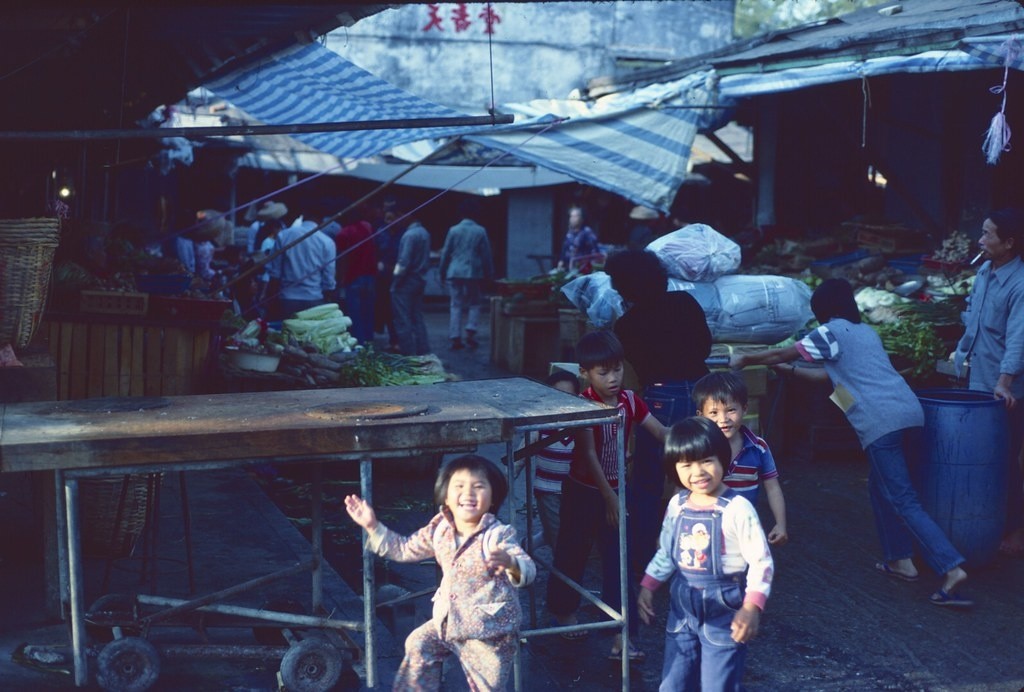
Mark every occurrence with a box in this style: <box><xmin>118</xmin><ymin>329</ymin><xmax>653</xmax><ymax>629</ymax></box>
<box><xmin>525</xmin><ymin>253</ymin><xmax>608</xmax><ymax>291</ymax></box>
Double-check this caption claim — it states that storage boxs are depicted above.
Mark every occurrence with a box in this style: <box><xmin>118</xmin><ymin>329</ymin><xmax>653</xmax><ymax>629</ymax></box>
<box><xmin>887</xmin><ymin>253</ymin><xmax>929</xmax><ymax>275</ymax></box>
<box><xmin>810</xmin><ymin>252</ymin><xmax>870</xmax><ymax>269</ymax></box>
<box><xmin>921</xmin><ymin>254</ymin><xmax>967</xmax><ymax>273</ymax></box>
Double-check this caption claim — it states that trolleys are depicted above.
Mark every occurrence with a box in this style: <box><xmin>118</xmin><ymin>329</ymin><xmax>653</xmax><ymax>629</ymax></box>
<box><xmin>701</xmin><ymin>358</ymin><xmax>781</xmax><ymax>453</ymax></box>
<box><xmin>1</xmin><ymin>380</ymin><xmax>630</xmax><ymax>692</ymax></box>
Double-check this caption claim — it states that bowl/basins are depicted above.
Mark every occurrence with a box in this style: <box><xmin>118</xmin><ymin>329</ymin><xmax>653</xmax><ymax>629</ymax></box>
<box><xmin>224</xmin><ymin>345</ymin><xmax>281</xmax><ymax>373</ymax></box>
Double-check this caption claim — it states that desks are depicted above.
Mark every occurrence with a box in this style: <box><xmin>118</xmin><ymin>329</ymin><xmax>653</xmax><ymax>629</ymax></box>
<box><xmin>0</xmin><ymin>378</ymin><xmax>630</xmax><ymax>692</ymax></box>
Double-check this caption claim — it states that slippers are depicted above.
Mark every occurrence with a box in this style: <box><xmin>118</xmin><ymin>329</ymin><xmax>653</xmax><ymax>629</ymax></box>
<box><xmin>549</xmin><ymin>620</ymin><xmax>588</xmax><ymax>640</ymax></box>
<box><xmin>608</xmin><ymin>647</ymin><xmax>644</xmax><ymax>661</ymax></box>
<box><xmin>880</xmin><ymin>562</ymin><xmax>919</xmax><ymax>581</ymax></box>
<box><xmin>929</xmin><ymin>589</ymin><xmax>973</xmax><ymax>606</ymax></box>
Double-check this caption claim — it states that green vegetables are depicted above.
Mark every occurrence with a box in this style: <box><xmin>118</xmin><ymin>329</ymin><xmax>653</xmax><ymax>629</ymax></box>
<box><xmin>280</xmin><ymin>303</ymin><xmax>359</xmax><ymax>353</ymax></box>
<box><xmin>859</xmin><ymin>316</ymin><xmax>948</xmax><ymax>381</ymax></box>
<box><xmin>343</xmin><ymin>357</ymin><xmax>456</xmax><ymax>388</ymax></box>
<box><xmin>221</xmin><ymin>311</ymin><xmax>279</xmax><ymax>352</ymax></box>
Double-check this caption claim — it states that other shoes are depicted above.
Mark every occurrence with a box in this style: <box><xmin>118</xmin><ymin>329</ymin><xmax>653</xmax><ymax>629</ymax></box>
<box><xmin>467</xmin><ymin>338</ymin><xmax>478</xmax><ymax>344</ymax></box>
<box><xmin>450</xmin><ymin>343</ymin><xmax>462</xmax><ymax>350</ymax></box>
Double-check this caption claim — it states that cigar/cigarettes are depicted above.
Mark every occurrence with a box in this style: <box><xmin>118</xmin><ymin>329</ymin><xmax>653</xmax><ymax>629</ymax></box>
<box><xmin>970</xmin><ymin>250</ymin><xmax>985</xmax><ymax>266</ymax></box>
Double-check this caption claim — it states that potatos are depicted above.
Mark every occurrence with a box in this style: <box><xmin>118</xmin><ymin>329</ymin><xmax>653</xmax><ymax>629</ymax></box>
<box><xmin>275</xmin><ymin>342</ymin><xmax>358</xmax><ymax>387</ymax></box>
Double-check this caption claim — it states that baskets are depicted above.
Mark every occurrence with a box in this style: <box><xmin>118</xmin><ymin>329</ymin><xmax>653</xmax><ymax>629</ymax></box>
<box><xmin>82</xmin><ymin>473</ymin><xmax>162</xmax><ymax>557</ymax></box>
<box><xmin>0</xmin><ymin>217</ymin><xmax>60</xmax><ymax>347</ymax></box>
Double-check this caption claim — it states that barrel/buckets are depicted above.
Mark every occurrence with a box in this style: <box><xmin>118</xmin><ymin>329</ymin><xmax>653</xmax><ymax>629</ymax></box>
<box><xmin>903</xmin><ymin>388</ymin><xmax>1013</xmax><ymax>571</ymax></box>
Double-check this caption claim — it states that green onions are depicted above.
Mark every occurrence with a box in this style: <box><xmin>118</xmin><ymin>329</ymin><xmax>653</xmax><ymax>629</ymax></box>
<box><xmin>870</xmin><ymin>302</ymin><xmax>963</xmax><ymax>323</ymax></box>
<box><xmin>376</xmin><ymin>352</ymin><xmax>438</xmax><ymax>374</ymax></box>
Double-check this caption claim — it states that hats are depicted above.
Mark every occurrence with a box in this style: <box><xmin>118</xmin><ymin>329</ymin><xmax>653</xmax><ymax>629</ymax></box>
<box><xmin>258</xmin><ymin>201</ymin><xmax>288</xmax><ymax>218</ymax></box>
<box><xmin>629</xmin><ymin>206</ymin><xmax>660</xmax><ymax>220</ymax></box>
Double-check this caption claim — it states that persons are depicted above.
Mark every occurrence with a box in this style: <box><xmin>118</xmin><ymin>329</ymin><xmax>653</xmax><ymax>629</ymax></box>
<box><xmin>691</xmin><ymin>369</ymin><xmax>792</xmax><ymax>547</ymax></box>
<box><xmin>333</xmin><ymin>205</ymin><xmax>379</xmax><ymax>350</ymax></box>
<box><xmin>235</xmin><ymin>165</ymin><xmax>341</xmax><ymax>330</ymax></box>
<box><xmin>626</xmin><ymin>203</ymin><xmax>661</xmax><ymax>246</ymax></box>
<box><xmin>952</xmin><ymin>206</ymin><xmax>1024</xmax><ymax>558</ymax></box>
<box><xmin>438</xmin><ymin>197</ymin><xmax>495</xmax><ymax>351</ymax></box>
<box><xmin>636</xmin><ymin>414</ymin><xmax>774</xmax><ymax>692</ymax></box>
<box><xmin>175</xmin><ymin>208</ymin><xmax>254</xmax><ymax>299</ymax></box>
<box><xmin>539</xmin><ymin>327</ymin><xmax>669</xmax><ymax>662</ymax></box>
<box><xmin>52</xmin><ymin>212</ymin><xmax>154</xmax><ymax>295</ymax></box>
<box><xmin>387</xmin><ymin>207</ymin><xmax>432</xmax><ymax>357</ymax></box>
<box><xmin>728</xmin><ymin>276</ymin><xmax>974</xmax><ymax>608</ymax></box>
<box><xmin>557</xmin><ymin>204</ymin><xmax>604</xmax><ymax>275</ymax></box>
<box><xmin>343</xmin><ymin>453</ymin><xmax>536</xmax><ymax>692</ymax></box>
<box><xmin>599</xmin><ymin>245</ymin><xmax>712</xmax><ymax>579</ymax></box>
<box><xmin>377</xmin><ymin>209</ymin><xmax>406</xmax><ymax>353</ymax></box>
<box><xmin>521</xmin><ymin>373</ymin><xmax>580</xmax><ymax>563</ymax></box>
